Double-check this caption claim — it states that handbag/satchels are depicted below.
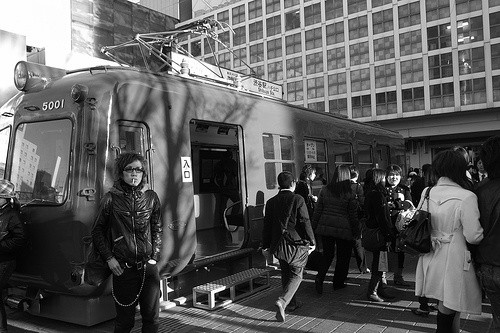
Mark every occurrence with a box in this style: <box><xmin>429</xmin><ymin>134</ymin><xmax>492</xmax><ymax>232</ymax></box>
<box><xmin>361</xmin><ymin>228</ymin><xmax>379</xmax><ymax>253</ymax></box>
<box><xmin>395</xmin><ymin>200</ymin><xmax>418</xmax><ymax>233</ymax></box>
<box><xmin>397</xmin><ymin>186</ymin><xmax>433</xmax><ymax>256</ymax></box>
<box><xmin>274</xmin><ymin>230</ymin><xmax>310</xmax><ymax>268</ymax></box>
<box><xmin>347</xmin><ymin>183</ymin><xmax>361</xmax><ymax>219</ymax></box>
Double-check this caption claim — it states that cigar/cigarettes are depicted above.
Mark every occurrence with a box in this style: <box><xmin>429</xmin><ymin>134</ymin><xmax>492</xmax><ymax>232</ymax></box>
<box><xmin>133</xmin><ymin>179</ymin><xmax>135</xmax><ymax>185</ymax></box>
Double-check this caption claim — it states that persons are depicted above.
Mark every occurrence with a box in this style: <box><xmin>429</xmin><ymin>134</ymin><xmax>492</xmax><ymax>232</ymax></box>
<box><xmin>91</xmin><ymin>152</ymin><xmax>163</xmax><ymax>333</ymax></box>
<box><xmin>263</xmin><ymin>171</ymin><xmax>316</xmax><ymax>323</ymax></box>
<box><xmin>361</xmin><ymin>168</ymin><xmax>394</xmax><ymax>302</ymax></box>
<box><xmin>311</xmin><ymin>165</ymin><xmax>362</xmax><ymax>294</ymax></box>
<box><xmin>455</xmin><ymin>148</ymin><xmax>488</xmax><ymax>183</ymax></box>
<box><xmin>294</xmin><ymin>162</ymin><xmax>437</xmax><ymax>289</ymax></box>
<box><xmin>0</xmin><ymin>179</ymin><xmax>26</xmax><ymax>333</ymax></box>
<box><xmin>465</xmin><ymin>135</ymin><xmax>500</xmax><ymax>333</ymax></box>
<box><xmin>415</xmin><ymin>150</ymin><xmax>484</xmax><ymax>333</ymax></box>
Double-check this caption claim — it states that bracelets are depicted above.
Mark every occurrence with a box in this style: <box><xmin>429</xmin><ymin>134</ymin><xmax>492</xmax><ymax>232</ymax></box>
<box><xmin>106</xmin><ymin>256</ymin><xmax>114</xmax><ymax>262</ymax></box>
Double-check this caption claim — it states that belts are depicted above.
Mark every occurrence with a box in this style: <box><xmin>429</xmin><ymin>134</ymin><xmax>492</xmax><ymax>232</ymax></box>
<box><xmin>121</xmin><ymin>258</ymin><xmax>150</xmax><ymax>272</ymax></box>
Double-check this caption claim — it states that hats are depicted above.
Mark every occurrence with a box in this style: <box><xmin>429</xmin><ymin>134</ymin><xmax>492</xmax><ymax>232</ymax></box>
<box><xmin>0</xmin><ymin>179</ymin><xmax>16</xmax><ymax>198</ymax></box>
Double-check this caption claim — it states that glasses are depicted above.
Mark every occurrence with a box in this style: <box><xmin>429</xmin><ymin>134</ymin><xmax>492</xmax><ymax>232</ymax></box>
<box><xmin>122</xmin><ymin>167</ymin><xmax>144</xmax><ymax>173</ymax></box>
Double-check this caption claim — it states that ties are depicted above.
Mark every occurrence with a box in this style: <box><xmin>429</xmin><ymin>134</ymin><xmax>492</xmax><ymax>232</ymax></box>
<box><xmin>482</xmin><ymin>175</ymin><xmax>485</xmax><ymax>180</ymax></box>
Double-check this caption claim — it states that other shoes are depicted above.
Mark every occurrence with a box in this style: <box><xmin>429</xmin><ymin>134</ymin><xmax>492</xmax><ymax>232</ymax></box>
<box><xmin>332</xmin><ymin>284</ymin><xmax>347</xmax><ymax>291</ymax></box>
<box><xmin>411</xmin><ymin>308</ymin><xmax>429</xmax><ymax>317</ymax></box>
<box><xmin>315</xmin><ymin>274</ymin><xmax>323</xmax><ymax>297</ymax></box>
<box><xmin>366</xmin><ymin>293</ymin><xmax>384</xmax><ymax>302</ymax></box>
<box><xmin>286</xmin><ymin>301</ymin><xmax>303</xmax><ymax>311</ymax></box>
<box><xmin>274</xmin><ymin>300</ymin><xmax>285</xmax><ymax>322</ymax></box>
<box><xmin>381</xmin><ymin>284</ymin><xmax>390</xmax><ymax>298</ymax></box>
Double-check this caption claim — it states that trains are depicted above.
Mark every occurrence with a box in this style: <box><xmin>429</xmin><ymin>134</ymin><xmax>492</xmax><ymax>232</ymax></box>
<box><xmin>0</xmin><ymin>20</ymin><xmax>406</xmax><ymax>326</ymax></box>
